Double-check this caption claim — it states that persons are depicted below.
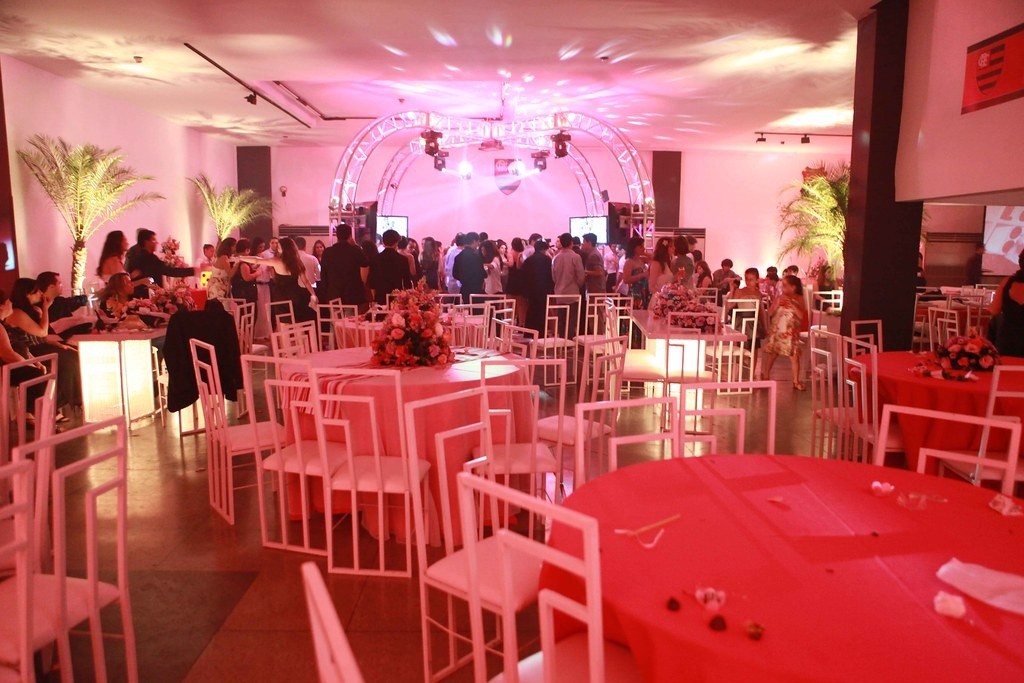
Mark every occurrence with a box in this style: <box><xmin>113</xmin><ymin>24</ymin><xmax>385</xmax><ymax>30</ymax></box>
<box><xmin>967</xmin><ymin>241</ymin><xmax>986</xmax><ymax>286</ymax></box>
<box><xmin>815</xmin><ymin>266</ymin><xmax>836</xmax><ymax>312</ymax></box>
<box><xmin>987</xmin><ymin>249</ymin><xmax>1024</xmax><ymax>357</ymax></box>
<box><xmin>0</xmin><ymin>223</ymin><xmax>807</xmax><ymax>433</ymax></box>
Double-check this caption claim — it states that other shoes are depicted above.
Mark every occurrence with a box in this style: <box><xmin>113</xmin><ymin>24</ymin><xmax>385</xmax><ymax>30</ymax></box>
<box><xmin>793</xmin><ymin>380</ymin><xmax>806</xmax><ymax>391</ymax></box>
<box><xmin>14</xmin><ymin>413</ymin><xmax>37</xmax><ymax>427</ymax></box>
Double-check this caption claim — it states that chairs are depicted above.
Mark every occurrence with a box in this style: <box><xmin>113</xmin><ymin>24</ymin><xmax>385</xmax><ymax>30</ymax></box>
<box><xmin>2</xmin><ymin>267</ymin><xmax>1024</xmax><ymax>683</ymax></box>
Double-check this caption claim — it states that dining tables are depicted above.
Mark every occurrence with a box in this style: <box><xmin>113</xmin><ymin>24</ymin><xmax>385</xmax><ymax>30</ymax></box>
<box><xmin>547</xmin><ymin>453</ymin><xmax>1024</xmax><ymax>683</ymax></box>
<box><xmin>335</xmin><ymin>314</ymin><xmax>485</xmax><ymax>353</ymax></box>
<box><xmin>848</xmin><ymin>351</ymin><xmax>1024</xmax><ymax>478</ymax></box>
<box><xmin>279</xmin><ymin>347</ymin><xmax>530</xmax><ymax>547</ymax></box>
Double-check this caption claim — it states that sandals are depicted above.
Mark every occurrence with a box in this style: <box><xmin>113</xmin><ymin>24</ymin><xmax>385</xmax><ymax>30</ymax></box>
<box><xmin>55</xmin><ymin>413</ymin><xmax>71</xmax><ymax>423</ymax></box>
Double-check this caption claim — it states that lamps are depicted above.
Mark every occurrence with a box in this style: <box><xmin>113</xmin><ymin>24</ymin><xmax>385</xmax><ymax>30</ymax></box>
<box><xmin>421</xmin><ymin>130</ymin><xmax>572</xmax><ymax>173</ymax></box>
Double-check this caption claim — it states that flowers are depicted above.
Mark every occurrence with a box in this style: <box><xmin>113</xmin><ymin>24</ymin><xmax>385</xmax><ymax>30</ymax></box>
<box><xmin>364</xmin><ymin>276</ymin><xmax>456</xmax><ymax>372</ymax></box>
<box><xmin>91</xmin><ymin>236</ymin><xmax>195</xmax><ymax>335</ymax></box>
<box><xmin>647</xmin><ymin>270</ymin><xmax>724</xmax><ymax>336</ymax></box>
<box><xmin>911</xmin><ymin>325</ymin><xmax>998</xmax><ymax>383</ymax></box>
